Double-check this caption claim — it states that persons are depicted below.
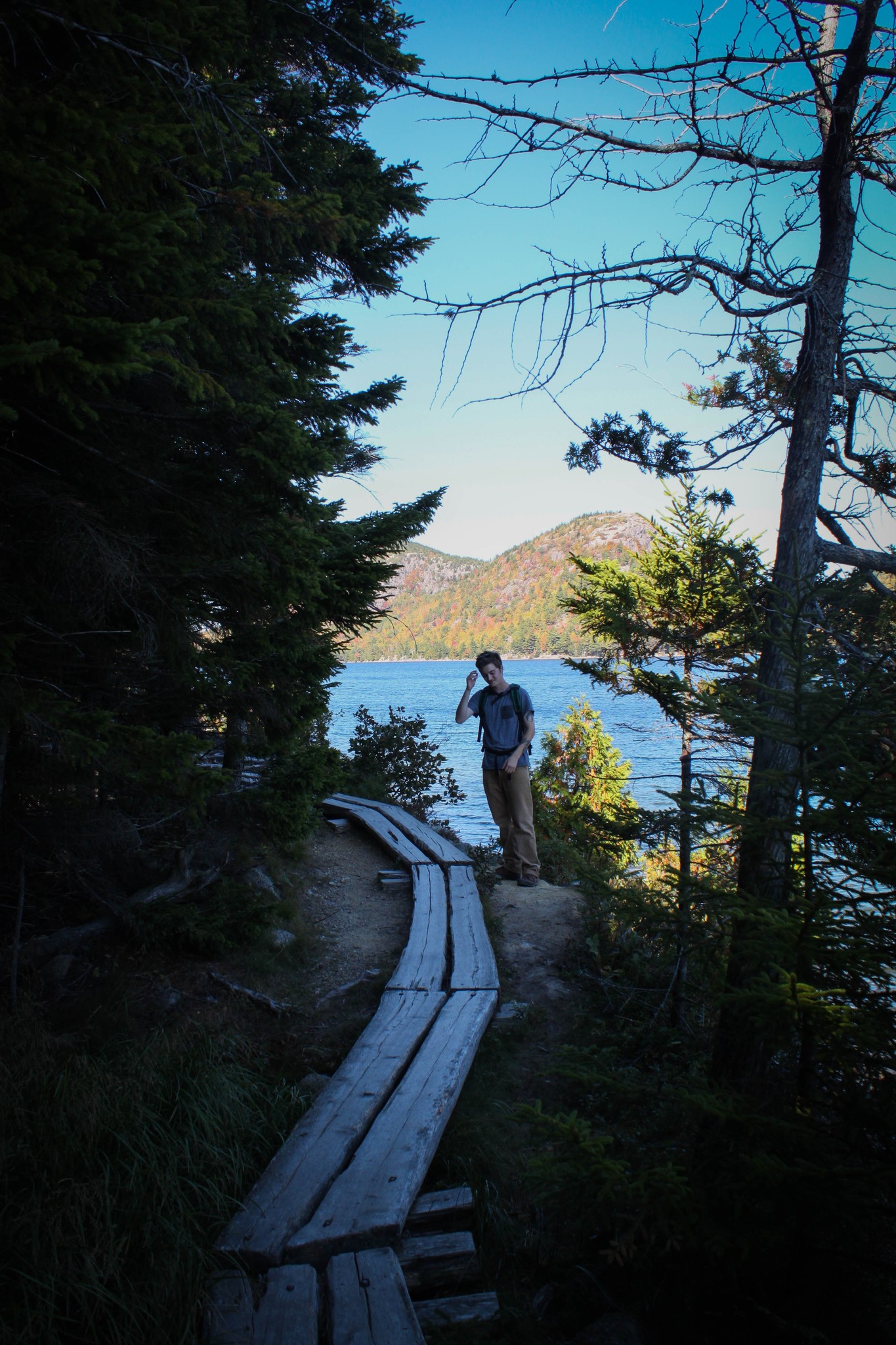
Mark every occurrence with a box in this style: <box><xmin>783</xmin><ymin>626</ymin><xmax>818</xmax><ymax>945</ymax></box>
<box><xmin>455</xmin><ymin>651</ymin><xmax>541</xmax><ymax>887</ymax></box>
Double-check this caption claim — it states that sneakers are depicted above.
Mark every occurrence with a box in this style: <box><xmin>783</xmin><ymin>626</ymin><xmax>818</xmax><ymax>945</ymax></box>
<box><xmin>495</xmin><ymin>866</ymin><xmax>518</xmax><ymax>881</ymax></box>
<box><xmin>518</xmin><ymin>874</ymin><xmax>539</xmax><ymax>887</ymax></box>
